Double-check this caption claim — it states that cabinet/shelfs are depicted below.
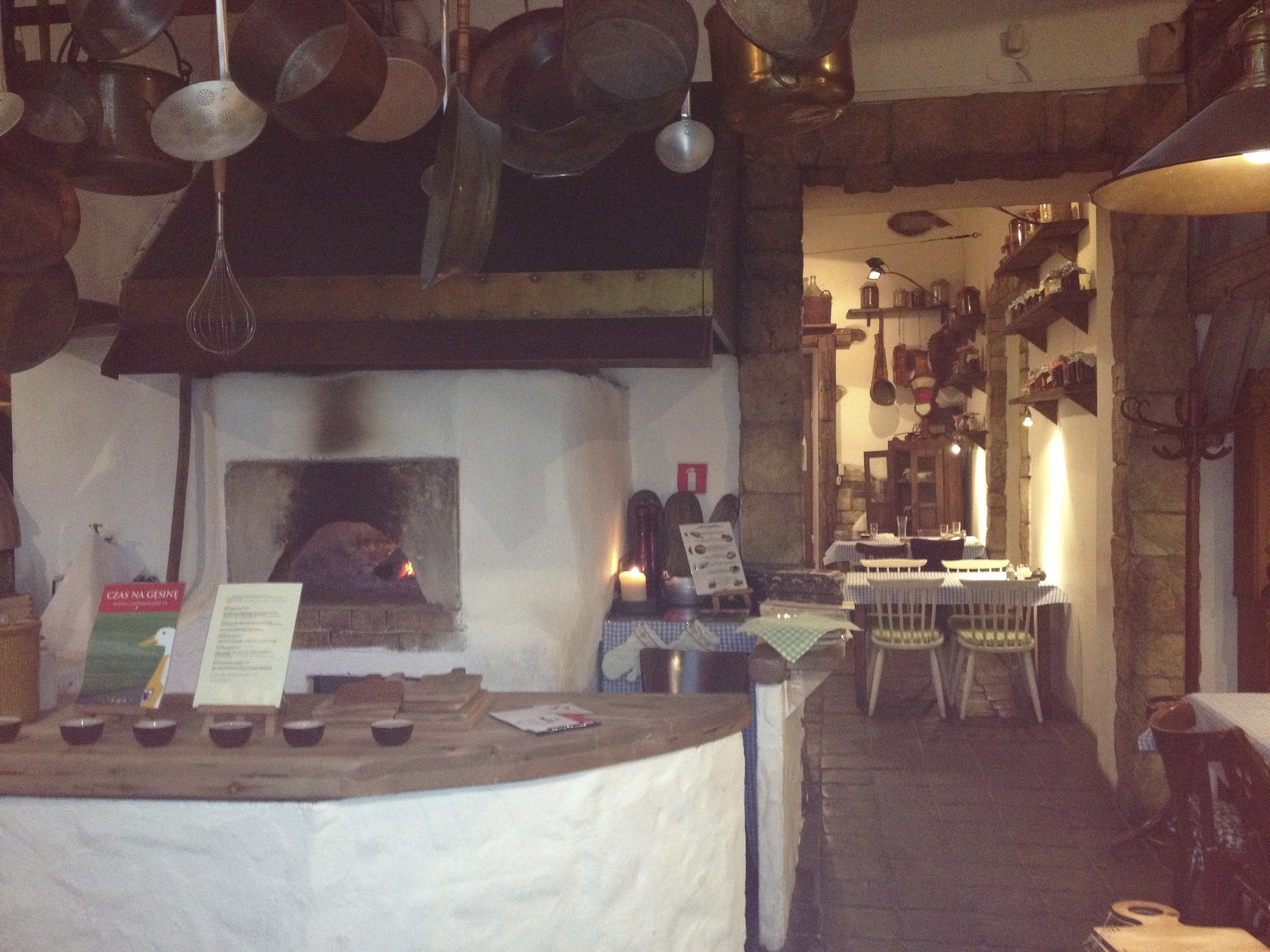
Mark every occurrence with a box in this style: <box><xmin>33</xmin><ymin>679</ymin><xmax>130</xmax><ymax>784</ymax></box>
<box><xmin>845</xmin><ymin>300</ymin><xmax>987</xmax><ymax>449</ymax></box>
<box><xmin>993</xmin><ymin>219</ymin><xmax>1097</xmax><ymax>426</ymax></box>
<box><xmin>859</xmin><ymin>438</ymin><xmax>964</xmax><ymax>532</ymax></box>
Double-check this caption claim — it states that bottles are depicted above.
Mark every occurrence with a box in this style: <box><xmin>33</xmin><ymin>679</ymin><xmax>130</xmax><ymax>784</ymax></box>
<box><xmin>1017</xmin><ymin>564</ymin><xmax>1026</xmax><ymax>581</ymax></box>
<box><xmin>1006</xmin><ymin>563</ymin><xmax>1015</xmax><ymax>581</ymax></box>
<box><xmin>961</xmin><ymin>529</ymin><xmax>966</xmax><ymax>542</ymax></box>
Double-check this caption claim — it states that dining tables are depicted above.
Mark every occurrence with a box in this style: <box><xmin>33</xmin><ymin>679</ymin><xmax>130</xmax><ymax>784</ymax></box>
<box><xmin>1189</xmin><ymin>690</ymin><xmax>1270</xmax><ymax>804</ymax></box>
<box><xmin>838</xmin><ymin>571</ymin><xmax>1069</xmax><ymax>709</ymax></box>
<box><xmin>822</xmin><ymin>535</ymin><xmax>989</xmax><ymax>573</ymax></box>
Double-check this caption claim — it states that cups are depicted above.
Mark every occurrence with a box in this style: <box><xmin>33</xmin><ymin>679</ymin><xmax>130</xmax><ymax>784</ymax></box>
<box><xmin>940</xmin><ymin>524</ymin><xmax>948</xmax><ymax>537</ymax></box>
<box><xmin>870</xmin><ymin>522</ymin><xmax>878</xmax><ymax>538</ymax></box>
<box><xmin>896</xmin><ymin>516</ymin><xmax>907</xmax><ymax>538</ymax></box>
<box><xmin>952</xmin><ymin>521</ymin><xmax>961</xmax><ymax>534</ymax></box>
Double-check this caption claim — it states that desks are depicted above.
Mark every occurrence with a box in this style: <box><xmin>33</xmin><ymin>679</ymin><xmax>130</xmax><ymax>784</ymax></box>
<box><xmin>1</xmin><ymin>695</ymin><xmax>746</xmax><ymax>950</ymax></box>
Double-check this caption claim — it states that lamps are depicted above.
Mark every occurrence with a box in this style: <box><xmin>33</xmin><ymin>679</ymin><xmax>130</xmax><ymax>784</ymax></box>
<box><xmin>951</xmin><ymin>439</ymin><xmax>962</xmax><ymax>455</ymax></box>
<box><xmin>866</xmin><ymin>257</ymin><xmax>886</xmax><ymax>281</ymax></box>
<box><xmin>1021</xmin><ymin>401</ymin><xmax>1036</xmax><ymax>428</ymax></box>
<box><xmin>1089</xmin><ymin>0</ymin><xmax>1268</xmax><ymax>221</ymax></box>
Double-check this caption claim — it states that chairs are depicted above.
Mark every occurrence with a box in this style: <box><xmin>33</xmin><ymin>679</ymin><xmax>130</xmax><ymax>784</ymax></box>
<box><xmin>866</xmin><ymin>577</ymin><xmax>953</xmax><ymax>720</ymax></box>
<box><xmin>940</xmin><ymin>557</ymin><xmax>1031</xmax><ymax>704</ymax></box>
<box><xmin>855</xmin><ymin>541</ymin><xmax>908</xmax><ymax>572</ymax></box>
<box><xmin>861</xmin><ymin>558</ymin><xmax>930</xmax><ymax>693</ymax></box>
<box><xmin>1147</xmin><ymin>700</ymin><xmax>1265</xmax><ymax>931</ymax></box>
<box><xmin>946</xmin><ymin>578</ymin><xmax>1045</xmax><ymax>723</ymax></box>
<box><xmin>910</xmin><ymin>538</ymin><xmax>965</xmax><ymax>572</ymax></box>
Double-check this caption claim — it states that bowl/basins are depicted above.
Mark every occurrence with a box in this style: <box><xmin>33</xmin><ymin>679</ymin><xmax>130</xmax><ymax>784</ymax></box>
<box><xmin>209</xmin><ymin>721</ymin><xmax>252</xmax><ymax>747</ymax></box>
<box><xmin>282</xmin><ymin>721</ymin><xmax>325</xmax><ymax>748</ymax></box>
<box><xmin>0</xmin><ymin>716</ymin><xmax>21</xmax><ymax>742</ymax></box>
<box><xmin>133</xmin><ymin>719</ymin><xmax>177</xmax><ymax>746</ymax></box>
<box><xmin>60</xmin><ymin>717</ymin><xmax>104</xmax><ymax>744</ymax></box>
<box><xmin>371</xmin><ymin>719</ymin><xmax>413</xmax><ymax>746</ymax></box>
